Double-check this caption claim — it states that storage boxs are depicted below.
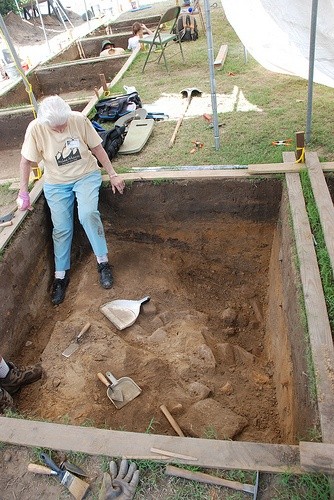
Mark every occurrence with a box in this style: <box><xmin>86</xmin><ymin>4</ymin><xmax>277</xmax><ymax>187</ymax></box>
<box><xmin>214</xmin><ymin>45</ymin><xmax>228</xmax><ymax>71</ymax></box>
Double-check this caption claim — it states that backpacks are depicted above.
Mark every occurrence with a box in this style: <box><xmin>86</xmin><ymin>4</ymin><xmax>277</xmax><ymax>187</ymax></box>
<box><xmin>173</xmin><ymin>15</ymin><xmax>199</xmax><ymax>42</ymax></box>
<box><xmin>95</xmin><ymin>125</ymin><xmax>127</xmax><ymax>167</ymax></box>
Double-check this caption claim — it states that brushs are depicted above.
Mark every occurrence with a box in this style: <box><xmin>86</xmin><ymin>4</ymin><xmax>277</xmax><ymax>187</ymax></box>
<box><xmin>39</xmin><ymin>453</ymin><xmax>90</xmax><ymax>500</ymax></box>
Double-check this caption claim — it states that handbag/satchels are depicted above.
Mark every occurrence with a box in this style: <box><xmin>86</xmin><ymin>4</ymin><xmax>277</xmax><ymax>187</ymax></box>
<box><xmin>123</xmin><ymin>86</ymin><xmax>139</xmax><ymax>97</ymax></box>
<box><xmin>95</xmin><ymin>91</ymin><xmax>142</xmax><ymax>123</ymax></box>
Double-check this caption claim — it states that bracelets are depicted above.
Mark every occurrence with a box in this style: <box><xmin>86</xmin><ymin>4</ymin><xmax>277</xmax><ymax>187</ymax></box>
<box><xmin>110</xmin><ymin>174</ymin><xmax>117</xmax><ymax>178</ymax></box>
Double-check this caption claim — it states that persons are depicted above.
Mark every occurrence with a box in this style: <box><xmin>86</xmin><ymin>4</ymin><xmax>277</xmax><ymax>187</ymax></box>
<box><xmin>100</xmin><ymin>40</ymin><xmax>125</xmax><ymax>56</ymax></box>
<box><xmin>128</xmin><ymin>22</ymin><xmax>152</xmax><ymax>50</ymax></box>
<box><xmin>15</xmin><ymin>96</ymin><xmax>125</xmax><ymax>305</ymax></box>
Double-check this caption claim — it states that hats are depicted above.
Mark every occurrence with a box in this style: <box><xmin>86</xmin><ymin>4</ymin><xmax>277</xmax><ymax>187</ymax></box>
<box><xmin>102</xmin><ymin>39</ymin><xmax>111</xmax><ymax>49</ymax></box>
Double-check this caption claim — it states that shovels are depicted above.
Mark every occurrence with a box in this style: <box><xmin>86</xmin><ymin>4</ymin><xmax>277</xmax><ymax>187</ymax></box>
<box><xmin>99</xmin><ymin>295</ymin><xmax>151</xmax><ymax>331</ymax></box>
<box><xmin>169</xmin><ymin>88</ymin><xmax>201</xmax><ymax>145</ymax></box>
<box><xmin>105</xmin><ymin>370</ymin><xmax>143</xmax><ymax>410</ymax></box>
<box><xmin>62</xmin><ymin>322</ymin><xmax>91</xmax><ymax>358</ymax></box>
<box><xmin>28</xmin><ymin>460</ymin><xmax>88</xmax><ymax>478</ymax></box>
<box><xmin>96</xmin><ymin>372</ymin><xmax>124</xmax><ymax>401</ymax></box>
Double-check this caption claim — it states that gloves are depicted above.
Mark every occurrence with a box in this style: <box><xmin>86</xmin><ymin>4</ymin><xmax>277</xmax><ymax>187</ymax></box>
<box><xmin>17</xmin><ymin>190</ymin><xmax>30</xmax><ymax>211</ymax></box>
<box><xmin>99</xmin><ymin>459</ymin><xmax>140</xmax><ymax>500</ymax></box>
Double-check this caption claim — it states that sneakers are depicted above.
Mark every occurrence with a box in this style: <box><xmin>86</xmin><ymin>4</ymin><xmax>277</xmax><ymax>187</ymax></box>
<box><xmin>0</xmin><ymin>388</ymin><xmax>14</xmax><ymax>413</ymax></box>
<box><xmin>97</xmin><ymin>261</ymin><xmax>114</xmax><ymax>288</ymax></box>
<box><xmin>51</xmin><ymin>273</ymin><xmax>69</xmax><ymax>305</ymax></box>
<box><xmin>0</xmin><ymin>361</ymin><xmax>42</xmax><ymax>396</ymax></box>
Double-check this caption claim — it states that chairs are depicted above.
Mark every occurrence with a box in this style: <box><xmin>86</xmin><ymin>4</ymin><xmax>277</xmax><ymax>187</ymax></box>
<box><xmin>138</xmin><ymin>6</ymin><xmax>184</xmax><ymax>74</ymax></box>
